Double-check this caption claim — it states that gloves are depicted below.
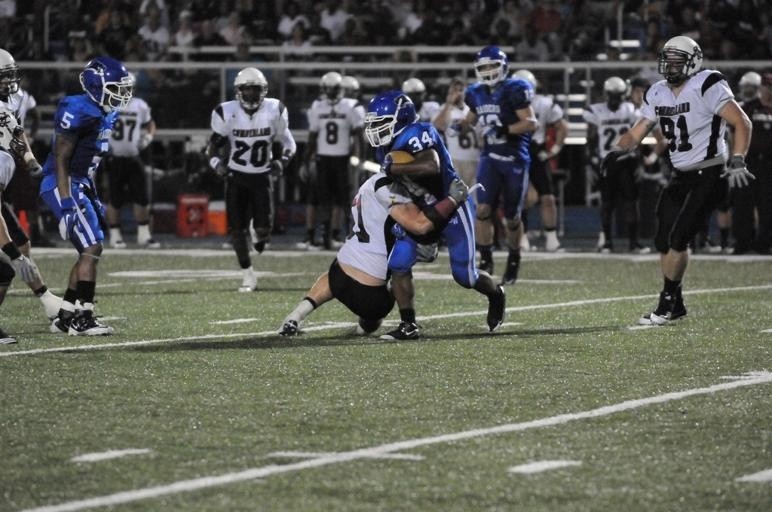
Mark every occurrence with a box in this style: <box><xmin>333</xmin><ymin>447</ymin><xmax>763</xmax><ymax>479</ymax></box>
<box><xmin>481</xmin><ymin>123</ymin><xmax>510</xmax><ymax>141</ymax></box>
<box><xmin>722</xmin><ymin>154</ymin><xmax>756</xmax><ymax>189</ymax></box>
<box><xmin>601</xmin><ymin>146</ymin><xmax>631</xmax><ymax>179</ymax></box>
<box><xmin>267</xmin><ymin>155</ymin><xmax>286</xmax><ymax>180</ymax></box>
<box><xmin>449</xmin><ymin>177</ymin><xmax>470</xmax><ymax>206</ymax></box>
<box><xmin>458</xmin><ymin>135</ymin><xmax>472</xmax><ymax>149</ymax></box>
<box><xmin>138</xmin><ymin>135</ymin><xmax>152</xmax><ymax>151</ymax></box>
<box><xmin>300</xmin><ymin>164</ymin><xmax>311</xmax><ymax>183</ymax></box>
<box><xmin>213</xmin><ymin>163</ymin><xmax>233</xmax><ymax>182</ymax></box>
<box><xmin>13</xmin><ymin>256</ymin><xmax>39</xmax><ymax>284</ymax></box>
<box><xmin>27</xmin><ymin>159</ymin><xmax>44</xmax><ymax>178</ymax></box>
<box><xmin>537</xmin><ymin>150</ymin><xmax>550</xmax><ymax>164</ymax></box>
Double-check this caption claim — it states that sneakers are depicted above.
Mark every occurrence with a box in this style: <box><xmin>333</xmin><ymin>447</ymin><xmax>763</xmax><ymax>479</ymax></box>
<box><xmin>652</xmin><ymin>292</ymin><xmax>678</xmax><ymax>328</ymax></box>
<box><xmin>545</xmin><ymin>241</ymin><xmax>559</xmax><ymax>253</ymax></box>
<box><xmin>50</xmin><ymin>310</ymin><xmax>75</xmax><ymax>334</ymax></box>
<box><xmin>137</xmin><ymin>237</ymin><xmax>161</xmax><ymax>250</ymax></box>
<box><xmin>66</xmin><ymin>310</ymin><xmax>114</xmax><ymax>336</ymax></box>
<box><xmin>379</xmin><ymin>322</ymin><xmax>421</xmax><ymax>341</ymax></box>
<box><xmin>108</xmin><ymin>238</ymin><xmax>126</xmax><ymax>250</ymax></box>
<box><xmin>294</xmin><ymin>238</ymin><xmax>313</xmax><ymax>253</ymax></box>
<box><xmin>239</xmin><ymin>278</ymin><xmax>259</xmax><ymax>295</ymax></box>
<box><xmin>520</xmin><ymin>242</ymin><xmax>531</xmax><ymax>253</ymax></box>
<box><xmin>476</xmin><ymin>260</ymin><xmax>494</xmax><ymax>276</ymax></box>
<box><xmin>599</xmin><ymin>241</ymin><xmax>612</xmax><ymax>253</ymax></box>
<box><xmin>248</xmin><ymin>218</ymin><xmax>267</xmax><ymax>254</ymax></box>
<box><xmin>276</xmin><ymin>317</ymin><xmax>300</xmax><ymax>337</ymax></box>
<box><xmin>501</xmin><ymin>256</ymin><xmax>519</xmax><ymax>285</ymax></box>
<box><xmin>638</xmin><ymin>299</ymin><xmax>690</xmax><ymax>325</ymax></box>
<box><xmin>486</xmin><ymin>285</ymin><xmax>506</xmax><ymax>331</ymax></box>
<box><xmin>629</xmin><ymin>241</ymin><xmax>652</xmax><ymax>255</ymax></box>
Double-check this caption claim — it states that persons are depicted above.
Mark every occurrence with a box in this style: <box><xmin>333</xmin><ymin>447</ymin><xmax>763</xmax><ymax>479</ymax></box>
<box><xmin>450</xmin><ymin>46</ymin><xmax>539</xmax><ymax>285</ymax></box>
<box><xmin>1</xmin><ymin>50</ymin><xmax>63</xmax><ymax>344</ymax></box>
<box><xmin>206</xmin><ymin>67</ymin><xmax>297</xmax><ymax>292</ymax></box>
<box><xmin>42</xmin><ymin>57</ymin><xmax>133</xmax><ymax>334</ymax></box>
<box><xmin>0</xmin><ymin>107</ymin><xmax>38</xmax><ymax>349</ymax></box>
<box><xmin>617</xmin><ymin>79</ymin><xmax>666</xmax><ymax>240</ymax></box>
<box><xmin>728</xmin><ymin>67</ymin><xmax>772</xmax><ymax>257</ymax></box>
<box><xmin>513</xmin><ymin>70</ymin><xmax>569</xmax><ymax>254</ymax></box>
<box><xmin>402</xmin><ymin>77</ymin><xmax>439</xmax><ymax>127</ymax></box>
<box><xmin>433</xmin><ymin>75</ymin><xmax>481</xmax><ymax>205</ymax></box>
<box><xmin>616</xmin><ymin>36</ymin><xmax>756</xmax><ymax>325</ymax></box>
<box><xmin>280</xmin><ymin>150</ymin><xmax>468</xmax><ymax>338</ymax></box>
<box><xmin>0</xmin><ymin>1</ymin><xmax>253</xmax><ymax>61</ymax></box>
<box><xmin>596</xmin><ymin>1</ymin><xmax>771</xmax><ymax>59</ymax></box>
<box><xmin>583</xmin><ymin>77</ymin><xmax>664</xmax><ymax>255</ymax></box>
<box><xmin>364</xmin><ymin>90</ymin><xmax>507</xmax><ymax>340</ymax></box>
<box><xmin>693</xmin><ymin>130</ymin><xmax>731</xmax><ymax>255</ymax></box>
<box><xmin>728</xmin><ymin>71</ymin><xmax>761</xmax><ymax>107</ymax></box>
<box><xmin>251</xmin><ymin>2</ymin><xmax>593</xmax><ymax>64</ymax></box>
<box><xmin>106</xmin><ymin>73</ymin><xmax>159</xmax><ymax>250</ymax></box>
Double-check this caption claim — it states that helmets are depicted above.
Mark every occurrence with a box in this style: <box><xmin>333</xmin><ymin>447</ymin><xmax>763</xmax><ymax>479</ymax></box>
<box><xmin>658</xmin><ymin>36</ymin><xmax>704</xmax><ymax>83</ymax></box>
<box><xmin>740</xmin><ymin>72</ymin><xmax>764</xmax><ymax>91</ymax></box>
<box><xmin>603</xmin><ymin>77</ymin><xmax>627</xmax><ymax>98</ymax></box>
<box><xmin>342</xmin><ymin>76</ymin><xmax>360</xmax><ymax>97</ymax></box>
<box><xmin>0</xmin><ymin>48</ymin><xmax>21</xmax><ymax>96</ymax></box>
<box><xmin>319</xmin><ymin>73</ymin><xmax>346</xmax><ymax>106</ymax></box>
<box><xmin>118</xmin><ymin>72</ymin><xmax>135</xmax><ymax>90</ymax></box>
<box><xmin>79</xmin><ymin>56</ymin><xmax>135</xmax><ymax>115</ymax></box>
<box><xmin>364</xmin><ymin>88</ymin><xmax>418</xmax><ymax>149</ymax></box>
<box><xmin>474</xmin><ymin>47</ymin><xmax>510</xmax><ymax>86</ymax></box>
<box><xmin>402</xmin><ymin>76</ymin><xmax>427</xmax><ymax>103</ymax></box>
<box><xmin>236</xmin><ymin>68</ymin><xmax>268</xmax><ymax>108</ymax></box>
<box><xmin>383</xmin><ymin>150</ymin><xmax>416</xmax><ymax>166</ymax></box>
<box><xmin>511</xmin><ymin>69</ymin><xmax>539</xmax><ymax>98</ymax></box>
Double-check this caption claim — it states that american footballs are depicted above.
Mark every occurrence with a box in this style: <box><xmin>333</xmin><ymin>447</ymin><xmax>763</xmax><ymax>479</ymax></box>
<box><xmin>385</xmin><ymin>151</ymin><xmax>415</xmax><ymax>164</ymax></box>
